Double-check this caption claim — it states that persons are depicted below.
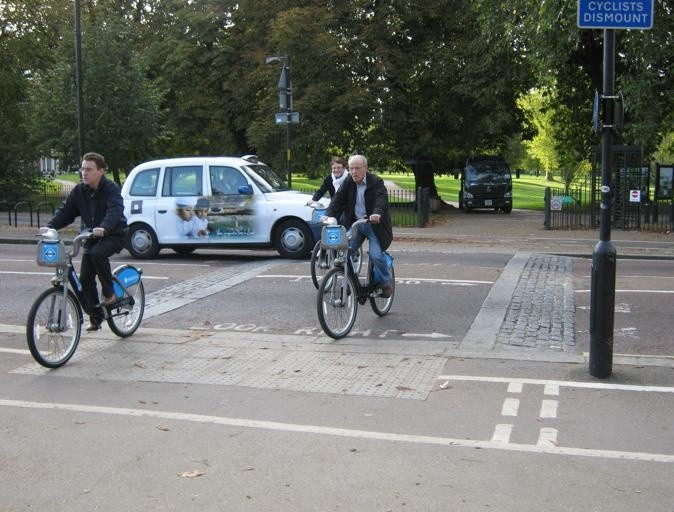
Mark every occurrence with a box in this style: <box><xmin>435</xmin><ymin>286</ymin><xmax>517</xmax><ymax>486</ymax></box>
<box><xmin>306</xmin><ymin>154</ymin><xmax>395</xmax><ymax>298</ymax></box>
<box><xmin>174</xmin><ymin>198</ymin><xmax>210</xmax><ymax>238</ymax></box>
<box><xmin>44</xmin><ymin>152</ymin><xmax>130</xmax><ymax>332</ymax></box>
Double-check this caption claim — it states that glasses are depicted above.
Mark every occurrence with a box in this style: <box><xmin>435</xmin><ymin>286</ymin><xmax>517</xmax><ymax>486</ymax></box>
<box><xmin>78</xmin><ymin>168</ymin><xmax>97</xmax><ymax>173</ymax></box>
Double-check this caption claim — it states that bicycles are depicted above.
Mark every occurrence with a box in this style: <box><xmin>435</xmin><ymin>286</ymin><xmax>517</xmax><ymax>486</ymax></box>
<box><xmin>311</xmin><ymin>210</ymin><xmax>397</xmax><ymax>340</ymax></box>
<box><xmin>303</xmin><ymin>198</ymin><xmax>365</xmax><ymax>293</ymax></box>
<box><xmin>23</xmin><ymin>227</ymin><xmax>149</xmax><ymax>373</ymax></box>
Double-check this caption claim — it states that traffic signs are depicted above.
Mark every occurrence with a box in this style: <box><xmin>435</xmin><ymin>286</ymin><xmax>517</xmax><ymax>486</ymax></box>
<box><xmin>577</xmin><ymin>1</ymin><xmax>654</xmax><ymax>31</ymax></box>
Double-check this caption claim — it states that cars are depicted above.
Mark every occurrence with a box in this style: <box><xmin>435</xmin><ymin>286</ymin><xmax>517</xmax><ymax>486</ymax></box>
<box><xmin>119</xmin><ymin>154</ymin><xmax>329</xmax><ymax>262</ymax></box>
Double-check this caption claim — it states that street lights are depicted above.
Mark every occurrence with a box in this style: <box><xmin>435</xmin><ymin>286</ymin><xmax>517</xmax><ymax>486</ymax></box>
<box><xmin>264</xmin><ymin>53</ymin><xmax>295</xmax><ymax>187</ymax></box>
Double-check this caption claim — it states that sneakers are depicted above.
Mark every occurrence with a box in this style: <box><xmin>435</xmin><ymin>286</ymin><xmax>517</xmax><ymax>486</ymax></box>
<box><xmin>352</xmin><ymin>249</ymin><xmax>360</xmax><ymax>263</ymax></box>
<box><xmin>382</xmin><ymin>284</ymin><xmax>394</xmax><ymax>298</ymax></box>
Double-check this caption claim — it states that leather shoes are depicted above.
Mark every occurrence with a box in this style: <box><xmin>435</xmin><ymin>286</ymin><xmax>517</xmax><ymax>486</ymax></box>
<box><xmin>86</xmin><ymin>322</ymin><xmax>101</xmax><ymax>331</ymax></box>
<box><xmin>101</xmin><ymin>293</ymin><xmax>117</xmax><ymax>306</ymax></box>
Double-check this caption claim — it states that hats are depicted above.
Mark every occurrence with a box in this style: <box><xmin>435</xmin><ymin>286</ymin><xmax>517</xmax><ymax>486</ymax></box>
<box><xmin>175</xmin><ymin>198</ymin><xmax>195</xmax><ymax>207</ymax></box>
<box><xmin>195</xmin><ymin>199</ymin><xmax>212</xmax><ymax>208</ymax></box>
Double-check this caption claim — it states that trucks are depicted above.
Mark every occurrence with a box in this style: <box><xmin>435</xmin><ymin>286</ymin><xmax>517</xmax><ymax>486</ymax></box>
<box><xmin>456</xmin><ymin>151</ymin><xmax>515</xmax><ymax>216</ymax></box>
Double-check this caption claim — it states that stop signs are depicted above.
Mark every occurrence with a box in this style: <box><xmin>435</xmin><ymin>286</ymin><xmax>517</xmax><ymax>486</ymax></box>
<box><xmin>631</xmin><ymin>191</ymin><xmax>638</xmax><ymax>197</ymax></box>
